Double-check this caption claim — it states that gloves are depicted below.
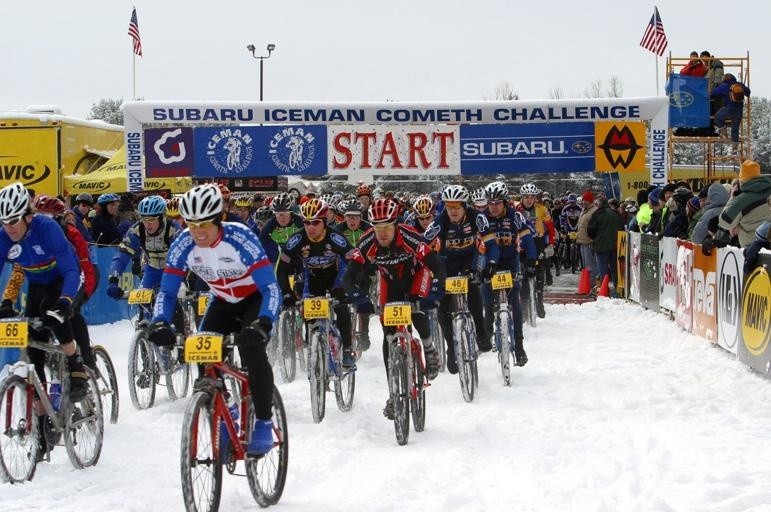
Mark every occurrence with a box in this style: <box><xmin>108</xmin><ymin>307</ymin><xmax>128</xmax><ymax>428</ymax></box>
<box><xmin>560</xmin><ymin>230</ymin><xmax>566</xmax><ymax>239</ymax></box>
<box><xmin>145</xmin><ymin>321</ymin><xmax>176</xmax><ymax>348</ymax></box>
<box><xmin>424</xmin><ymin>298</ymin><xmax>439</xmax><ymax>309</ymax></box>
<box><xmin>281</xmin><ymin>288</ymin><xmax>299</xmax><ymax>305</ymax></box>
<box><xmin>542</xmin><ymin>245</ymin><xmax>554</xmax><ymax>260</ymax></box>
<box><xmin>41</xmin><ymin>297</ymin><xmax>72</xmax><ymax>327</ymax></box>
<box><xmin>702</xmin><ymin>240</ymin><xmax>713</xmax><ymax>256</ymax></box>
<box><xmin>525</xmin><ymin>260</ymin><xmax>537</xmax><ymax>279</ymax></box>
<box><xmin>482</xmin><ymin>262</ymin><xmax>497</xmax><ymax>280</ymax></box>
<box><xmin>348</xmin><ymin>289</ymin><xmax>362</xmax><ymax>301</ymax></box>
<box><xmin>107</xmin><ymin>276</ymin><xmax>124</xmax><ymax>300</ymax></box>
<box><xmin>715</xmin><ymin>229</ymin><xmax>729</xmax><ymax>247</ymax></box>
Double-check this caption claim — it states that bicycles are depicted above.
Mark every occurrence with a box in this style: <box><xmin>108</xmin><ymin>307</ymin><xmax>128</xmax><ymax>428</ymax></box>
<box><xmin>162</xmin><ymin>328</ymin><xmax>291</xmax><ymax>512</ymax></box>
<box><xmin>288</xmin><ymin>295</ymin><xmax>359</xmax><ymax>427</ymax></box>
<box><xmin>357</xmin><ymin>296</ymin><xmax>434</xmax><ymax>446</ymax></box>
<box><xmin>86</xmin><ymin>345</ymin><xmax>127</xmax><ymax>423</ymax></box>
<box><xmin>119</xmin><ymin>290</ymin><xmax>193</xmax><ymax>413</ymax></box>
<box><xmin>0</xmin><ymin>313</ymin><xmax>106</xmax><ymax>487</ymax></box>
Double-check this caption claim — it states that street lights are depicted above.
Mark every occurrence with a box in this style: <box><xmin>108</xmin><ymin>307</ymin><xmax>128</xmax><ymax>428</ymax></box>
<box><xmin>244</xmin><ymin>41</ymin><xmax>276</xmax><ymax>101</ymax></box>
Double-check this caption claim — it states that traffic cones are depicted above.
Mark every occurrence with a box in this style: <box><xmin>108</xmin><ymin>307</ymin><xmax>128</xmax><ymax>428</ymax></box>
<box><xmin>595</xmin><ymin>274</ymin><xmax>610</xmax><ymax>298</ymax></box>
<box><xmin>575</xmin><ymin>266</ymin><xmax>590</xmax><ymax>296</ymax></box>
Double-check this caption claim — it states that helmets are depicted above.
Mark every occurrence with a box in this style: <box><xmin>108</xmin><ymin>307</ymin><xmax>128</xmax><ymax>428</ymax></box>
<box><xmin>74</xmin><ymin>193</ymin><xmax>94</xmax><ymax>206</ymax></box>
<box><xmin>0</xmin><ymin>183</ymin><xmax>33</xmax><ymax>219</ymax></box>
<box><xmin>36</xmin><ymin>197</ymin><xmax>65</xmax><ymax>218</ymax></box>
<box><xmin>137</xmin><ymin>183</ymin><xmax>539</xmax><ymax>222</ymax></box>
<box><xmin>98</xmin><ymin>193</ymin><xmax>122</xmax><ymax>207</ymax></box>
<box><xmin>539</xmin><ymin>192</ymin><xmax>584</xmax><ymax>206</ymax></box>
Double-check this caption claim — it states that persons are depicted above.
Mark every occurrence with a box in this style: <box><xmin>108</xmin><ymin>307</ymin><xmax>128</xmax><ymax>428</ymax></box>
<box><xmin>701</xmin><ymin>51</ymin><xmax>723</xmax><ymax>136</ymax></box>
<box><xmin>680</xmin><ymin>51</ymin><xmax>705</xmax><ymax>136</ymax></box>
<box><xmin>710</xmin><ymin>73</ymin><xmax>751</xmax><ymax>154</ymax></box>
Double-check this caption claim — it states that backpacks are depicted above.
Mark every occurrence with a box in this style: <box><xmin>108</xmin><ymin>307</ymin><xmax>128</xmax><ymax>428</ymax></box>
<box><xmin>236</xmin><ymin>316</ymin><xmax>272</xmax><ymax>344</ymax></box>
<box><xmin>726</xmin><ymin>82</ymin><xmax>743</xmax><ymax>103</ymax></box>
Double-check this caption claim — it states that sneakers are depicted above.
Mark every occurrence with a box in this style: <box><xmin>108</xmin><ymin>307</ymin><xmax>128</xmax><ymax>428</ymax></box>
<box><xmin>246</xmin><ymin>421</ymin><xmax>273</xmax><ymax>457</ymax></box>
<box><xmin>70</xmin><ymin>362</ymin><xmax>89</xmax><ymax>402</ymax></box>
<box><xmin>341</xmin><ymin>295</ymin><xmax>549</xmax><ymax>424</ymax></box>
<box><xmin>547</xmin><ymin>260</ymin><xmax>582</xmax><ymax>284</ymax></box>
<box><xmin>219</xmin><ymin>405</ymin><xmax>239</xmax><ymax>445</ymax></box>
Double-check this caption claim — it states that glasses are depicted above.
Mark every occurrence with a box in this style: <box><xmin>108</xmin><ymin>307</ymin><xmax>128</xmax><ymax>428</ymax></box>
<box><xmin>186</xmin><ymin>222</ymin><xmax>215</xmax><ymax>228</ymax></box>
<box><xmin>0</xmin><ymin>218</ymin><xmax>22</xmax><ymax>226</ymax></box>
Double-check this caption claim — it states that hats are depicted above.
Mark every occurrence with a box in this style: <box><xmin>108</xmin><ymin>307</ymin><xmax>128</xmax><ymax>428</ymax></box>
<box><xmin>583</xmin><ymin>192</ymin><xmax>638</xmax><ymax>215</ymax></box>
<box><xmin>755</xmin><ymin>221</ymin><xmax>771</xmax><ymax>244</ymax></box>
<box><xmin>700</xmin><ymin>51</ymin><xmax>710</xmax><ymax>57</ymax></box>
<box><xmin>737</xmin><ymin>160</ymin><xmax>761</xmax><ymax>181</ymax></box>
<box><xmin>639</xmin><ymin>183</ymin><xmax>701</xmax><ymax>212</ymax></box>
<box><xmin>707</xmin><ymin>216</ymin><xmax>719</xmax><ymax>232</ymax></box>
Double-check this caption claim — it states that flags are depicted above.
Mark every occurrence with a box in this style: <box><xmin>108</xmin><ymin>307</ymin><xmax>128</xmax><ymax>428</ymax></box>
<box><xmin>640</xmin><ymin>7</ymin><xmax>667</xmax><ymax>57</ymax></box>
<box><xmin>128</xmin><ymin>8</ymin><xmax>142</xmax><ymax>57</ymax></box>
